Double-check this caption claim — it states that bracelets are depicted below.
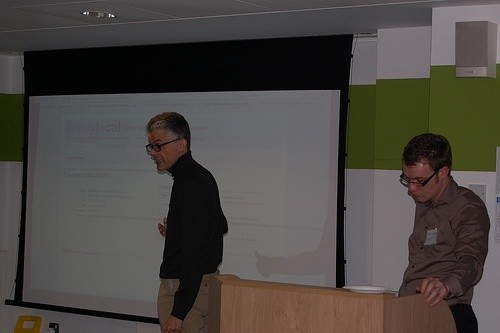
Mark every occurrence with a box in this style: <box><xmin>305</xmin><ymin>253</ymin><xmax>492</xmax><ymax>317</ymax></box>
<box><xmin>439</xmin><ymin>279</ymin><xmax>448</xmax><ymax>293</ymax></box>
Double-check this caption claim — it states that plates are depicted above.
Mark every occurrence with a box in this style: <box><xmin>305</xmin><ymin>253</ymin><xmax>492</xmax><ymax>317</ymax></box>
<box><xmin>343</xmin><ymin>286</ymin><xmax>390</xmax><ymax>293</ymax></box>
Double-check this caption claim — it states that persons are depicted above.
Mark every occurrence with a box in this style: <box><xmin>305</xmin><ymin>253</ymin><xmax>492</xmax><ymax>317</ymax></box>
<box><xmin>399</xmin><ymin>133</ymin><xmax>490</xmax><ymax>333</ymax></box>
<box><xmin>145</xmin><ymin>112</ymin><xmax>229</xmax><ymax>333</ymax></box>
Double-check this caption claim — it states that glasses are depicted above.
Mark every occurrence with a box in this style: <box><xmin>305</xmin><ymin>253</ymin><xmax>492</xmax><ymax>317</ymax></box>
<box><xmin>145</xmin><ymin>138</ymin><xmax>181</xmax><ymax>152</ymax></box>
<box><xmin>400</xmin><ymin>172</ymin><xmax>436</xmax><ymax>187</ymax></box>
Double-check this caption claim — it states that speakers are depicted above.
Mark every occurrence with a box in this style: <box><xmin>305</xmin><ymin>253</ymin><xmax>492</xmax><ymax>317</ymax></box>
<box><xmin>455</xmin><ymin>21</ymin><xmax>498</xmax><ymax>79</ymax></box>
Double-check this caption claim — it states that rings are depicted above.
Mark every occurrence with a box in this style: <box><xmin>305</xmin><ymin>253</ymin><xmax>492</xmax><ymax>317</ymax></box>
<box><xmin>434</xmin><ymin>287</ymin><xmax>440</xmax><ymax>291</ymax></box>
